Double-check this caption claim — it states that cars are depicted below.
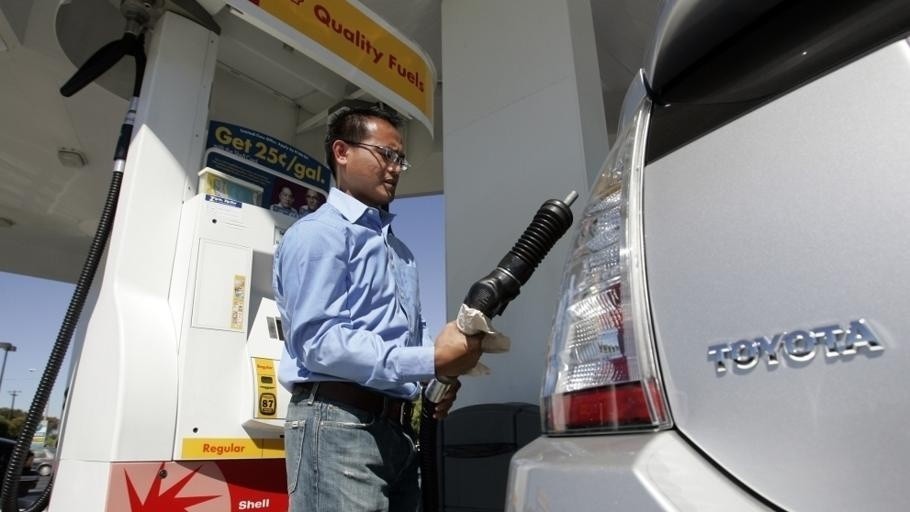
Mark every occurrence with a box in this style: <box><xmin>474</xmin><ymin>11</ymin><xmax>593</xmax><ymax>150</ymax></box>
<box><xmin>30</xmin><ymin>449</ymin><xmax>56</xmax><ymax>476</ymax></box>
<box><xmin>504</xmin><ymin>0</ymin><xmax>909</xmax><ymax>512</ymax></box>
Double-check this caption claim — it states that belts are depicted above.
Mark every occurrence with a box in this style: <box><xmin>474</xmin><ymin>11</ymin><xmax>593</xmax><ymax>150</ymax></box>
<box><xmin>292</xmin><ymin>380</ymin><xmax>412</xmax><ymax>426</ymax></box>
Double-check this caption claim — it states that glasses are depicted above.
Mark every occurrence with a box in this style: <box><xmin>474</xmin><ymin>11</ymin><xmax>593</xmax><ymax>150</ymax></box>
<box><xmin>343</xmin><ymin>139</ymin><xmax>410</xmax><ymax>171</ymax></box>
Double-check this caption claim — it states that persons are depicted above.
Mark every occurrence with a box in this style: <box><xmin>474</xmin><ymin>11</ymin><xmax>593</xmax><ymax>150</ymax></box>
<box><xmin>272</xmin><ymin>98</ymin><xmax>486</xmax><ymax>512</ymax></box>
<box><xmin>269</xmin><ymin>187</ymin><xmax>300</xmax><ymax>219</ymax></box>
<box><xmin>299</xmin><ymin>188</ymin><xmax>321</xmax><ymax>219</ymax></box>
